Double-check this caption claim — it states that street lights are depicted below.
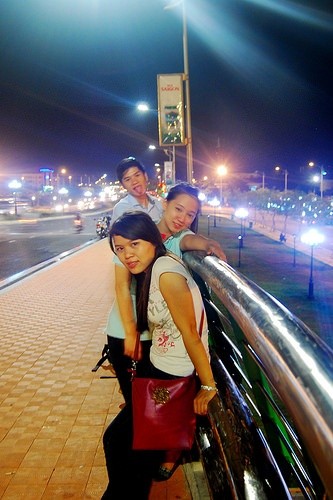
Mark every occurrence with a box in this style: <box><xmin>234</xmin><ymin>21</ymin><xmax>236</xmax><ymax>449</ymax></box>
<box><xmin>8</xmin><ymin>180</ymin><xmax>23</xmax><ymax>214</ymax></box>
<box><xmin>255</xmin><ymin>170</ymin><xmax>265</xmax><ymax>189</ymax></box>
<box><xmin>300</xmin><ymin>229</ymin><xmax>325</xmax><ymax>299</ymax></box>
<box><xmin>198</xmin><ymin>193</ymin><xmax>206</xmax><ymax>216</ymax></box>
<box><xmin>235</xmin><ymin>208</ymin><xmax>249</xmax><ymax>248</ymax></box>
<box><xmin>210</xmin><ymin>198</ymin><xmax>220</xmax><ymax>227</ymax></box>
<box><xmin>309</xmin><ymin>162</ymin><xmax>323</xmax><ymax>198</ymax></box>
<box><xmin>58</xmin><ymin>188</ymin><xmax>68</xmax><ymax>216</ymax></box>
<box><xmin>275</xmin><ymin>166</ymin><xmax>288</xmax><ymax>193</ymax></box>
<box><xmin>136</xmin><ymin>103</ymin><xmax>176</xmax><ymax>184</ymax></box>
<box><xmin>84</xmin><ymin>191</ymin><xmax>92</xmax><ymax>210</ymax></box>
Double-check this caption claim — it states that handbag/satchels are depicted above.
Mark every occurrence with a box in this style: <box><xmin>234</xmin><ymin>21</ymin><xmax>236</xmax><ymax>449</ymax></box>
<box><xmin>132</xmin><ymin>374</ymin><xmax>196</xmax><ymax>450</ymax></box>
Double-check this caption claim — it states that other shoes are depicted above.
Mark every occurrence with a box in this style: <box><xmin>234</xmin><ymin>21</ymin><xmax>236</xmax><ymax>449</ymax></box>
<box><xmin>154</xmin><ymin>450</ymin><xmax>185</xmax><ymax>481</ymax></box>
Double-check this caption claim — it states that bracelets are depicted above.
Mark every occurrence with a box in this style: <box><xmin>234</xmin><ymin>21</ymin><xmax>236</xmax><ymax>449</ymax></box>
<box><xmin>200</xmin><ymin>385</ymin><xmax>218</xmax><ymax>391</ymax></box>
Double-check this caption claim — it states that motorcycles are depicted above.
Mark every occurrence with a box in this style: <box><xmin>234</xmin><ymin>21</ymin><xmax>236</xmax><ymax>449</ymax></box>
<box><xmin>96</xmin><ymin>216</ymin><xmax>111</xmax><ymax>239</ymax></box>
<box><xmin>74</xmin><ymin>220</ymin><xmax>83</xmax><ymax>234</ymax></box>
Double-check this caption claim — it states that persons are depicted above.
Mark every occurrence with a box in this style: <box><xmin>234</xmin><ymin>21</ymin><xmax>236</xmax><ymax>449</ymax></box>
<box><xmin>74</xmin><ymin>215</ymin><xmax>82</xmax><ymax>229</ymax></box>
<box><xmin>111</xmin><ymin>157</ymin><xmax>166</xmax><ymax>226</ymax></box>
<box><xmin>100</xmin><ymin>210</ymin><xmax>219</xmax><ymax>500</ymax></box>
<box><xmin>105</xmin><ymin>182</ymin><xmax>227</xmax><ymax>480</ymax></box>
